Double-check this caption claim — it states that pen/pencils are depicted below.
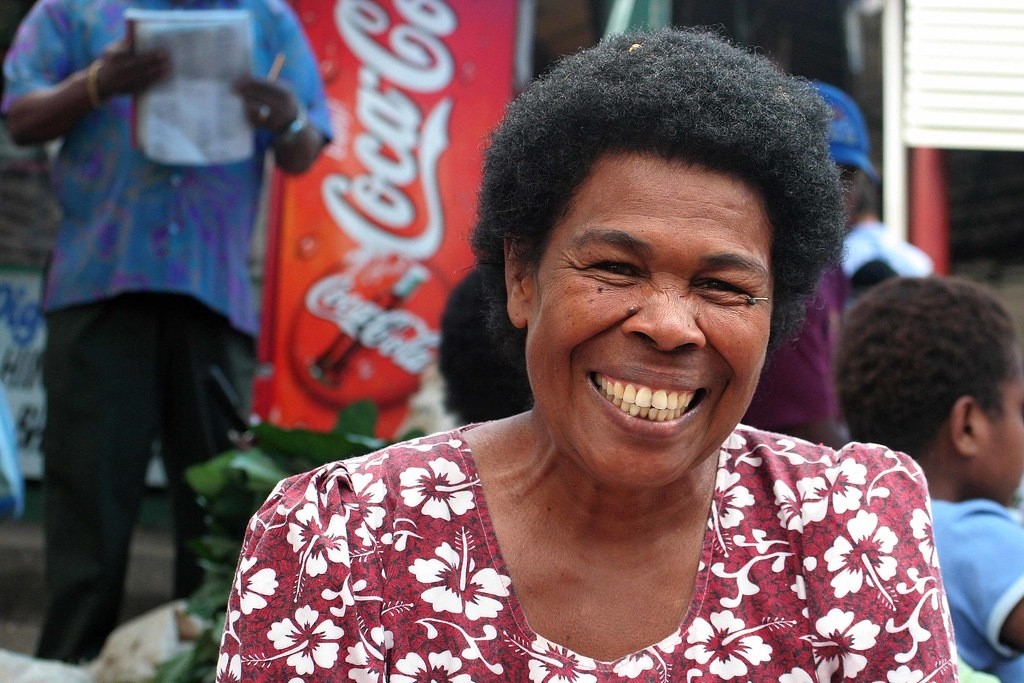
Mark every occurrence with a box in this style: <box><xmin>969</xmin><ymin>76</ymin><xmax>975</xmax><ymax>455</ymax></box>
<box><xmin>266</xmin><ymin>51</ymin><xmax>285</xmax><ymax>82</ymax></box>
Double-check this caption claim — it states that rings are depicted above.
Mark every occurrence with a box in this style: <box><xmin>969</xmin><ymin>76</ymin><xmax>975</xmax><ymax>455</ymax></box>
<box><xmin>259</xmin><ymin>105</ymin><xmax>270</xmax><ymax>122</ymax></box>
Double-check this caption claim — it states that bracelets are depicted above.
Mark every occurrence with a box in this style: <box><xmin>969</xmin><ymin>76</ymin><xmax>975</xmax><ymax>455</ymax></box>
<box><xmin>87</xmin><ymin>60</ymin><xmax>104</xmax><ymax>108</ymax></box>
<box><xmin>279</xmin><ymin>113</ymin><xmax>309</xmax><ymax>147</ymax></box>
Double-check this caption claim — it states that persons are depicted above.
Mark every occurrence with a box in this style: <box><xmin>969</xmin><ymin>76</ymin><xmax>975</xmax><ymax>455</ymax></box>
<box><xmin>744</xmin><ymin>75</ymin><xmax>1024</xmax><ymax>683</ymax></box>
<box><xmin>0</xmin><ymin>0</ymin><xmax>334</xmax><ymax>664</ymax></box>
<box><xmin>216</xmin><ymin>29</ymin><xmax>957</xmax><ymax>683</ymax></box>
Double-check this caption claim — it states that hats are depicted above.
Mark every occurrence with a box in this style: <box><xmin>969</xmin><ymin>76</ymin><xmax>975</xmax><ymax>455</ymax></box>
<box><xmin>806</xmin><ymin>81</ymin><xmax>876</xmax><ymax>180</ymax></box>
<box><xmin>839</xmin><ymin>225</ymin><xmax>934</xmax><ymax>280</ymax></box>
